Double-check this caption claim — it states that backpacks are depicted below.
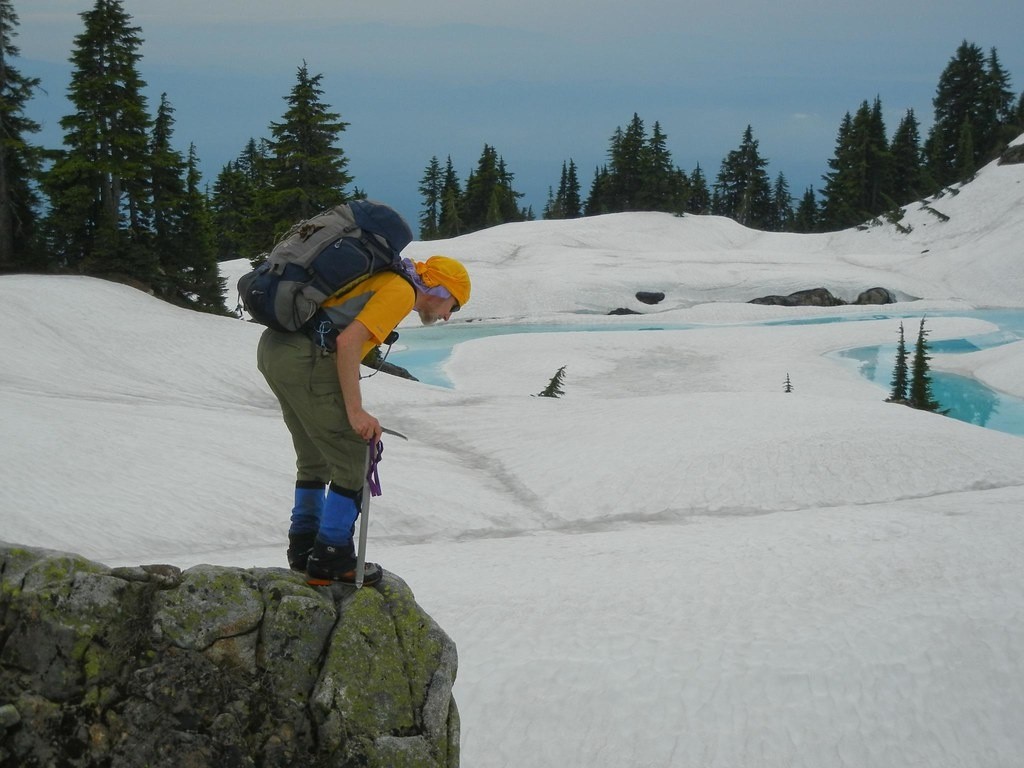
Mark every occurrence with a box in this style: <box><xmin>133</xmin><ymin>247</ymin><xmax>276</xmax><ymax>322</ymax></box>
<box><xmin>239</xmin><ymin>198</ymin><xmax>414</xmax><ymax>334</ymax></box>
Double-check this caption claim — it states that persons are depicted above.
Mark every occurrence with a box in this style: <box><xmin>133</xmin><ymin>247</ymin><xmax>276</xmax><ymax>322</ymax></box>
<box><xmin>257</xmin><ymin>255</ymin><xmax>471</xmax><ymax>585</ymax></box>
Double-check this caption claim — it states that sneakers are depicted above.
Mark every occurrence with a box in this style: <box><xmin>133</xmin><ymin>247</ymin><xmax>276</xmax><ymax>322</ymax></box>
<box><xmin>306</xmin><ymin>554</ymin><xmax>382</xmax><ymax>587</ymax></box>
<box><xmin>286</xmin><ymin>546</ymin><xmax>315</xmax><ymax>573</ymax></box>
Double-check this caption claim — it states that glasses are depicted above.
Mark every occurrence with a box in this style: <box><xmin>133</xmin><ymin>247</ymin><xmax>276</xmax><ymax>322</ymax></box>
<box><xmin>450</xmin><ymin>303</ymin><xmax>460</xmax><ymax>312</ymax></box>
